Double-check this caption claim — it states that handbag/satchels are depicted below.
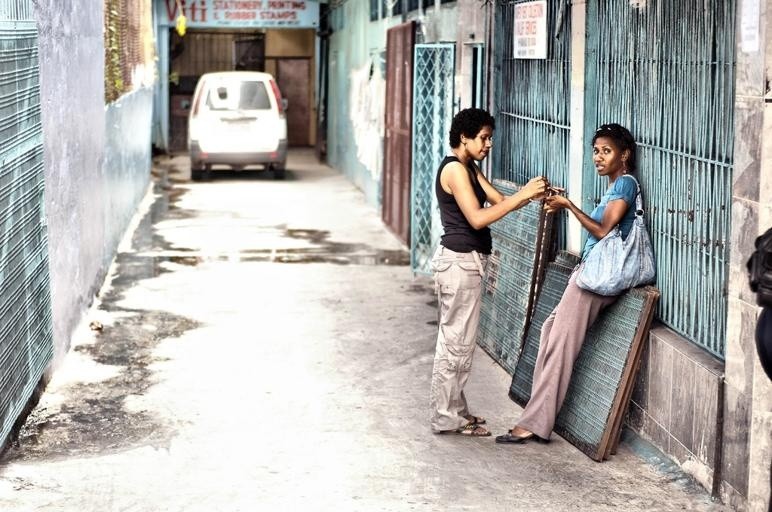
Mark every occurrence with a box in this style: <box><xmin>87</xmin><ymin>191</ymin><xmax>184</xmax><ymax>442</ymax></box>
<box><xmin>575</xmin><ymin>173</ymin><xmax>657</xmax><ymax>297</ymax></box>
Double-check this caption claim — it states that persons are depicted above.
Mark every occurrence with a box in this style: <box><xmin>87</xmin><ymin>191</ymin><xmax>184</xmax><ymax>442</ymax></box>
<box><xmin>430</xmin><ymin>107</ymin><xmax>568</xmax><ymax>438</ymax></box>
<box><xmin>496</xmin><ymin>124</ymin><xmax>644</xmax><ymax>444</ymax></box>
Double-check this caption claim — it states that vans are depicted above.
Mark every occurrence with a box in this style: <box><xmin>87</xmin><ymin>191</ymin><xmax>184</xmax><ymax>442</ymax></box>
<box><xmin>180</xmin><ymin>70</ymin><xmax>290</xmax><ymax>182</ymax></box>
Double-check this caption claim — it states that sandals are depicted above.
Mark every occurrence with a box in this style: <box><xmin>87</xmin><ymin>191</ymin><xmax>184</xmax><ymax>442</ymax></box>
<box><xmin>432</xmin><ymin>413</ymin><xmax>492</xmax><ymax>437</ymax></box>
<box><xmin>495</xmin><ymin>428</ymin><xmax>551</xmax><ymax>445</ymax></box>
